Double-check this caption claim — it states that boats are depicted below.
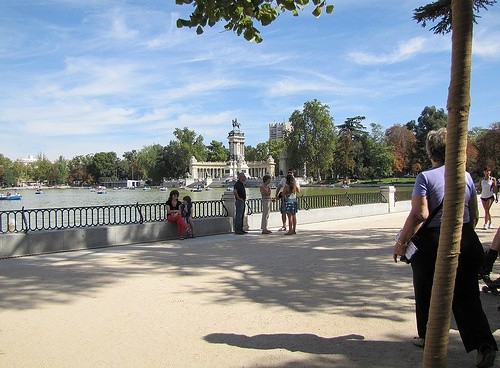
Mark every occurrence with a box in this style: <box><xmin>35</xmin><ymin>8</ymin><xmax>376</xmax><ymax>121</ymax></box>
<box><xmin>0</xmin><ymin>191</ymin><xmax>23</xmax><ymax>200</ymax></box>
<box><xmin>160</xmin><ymin>186</ymin><xmax>168</xmax><ymax>191</ymax></box>
<box><xmin>88</xmin><ymin>185</ymin><xmax>136</xmax><ymax>194</ymax></box>
<box><xmin>34</xmin><ymin>190</ymin><xmax>45</xmax><ymax>194</ymax></box>
<box><xmin>328</xmin><ymin>183</ymin><xmax>336</xmax><ymax>188</ymax></box>
<box><xmin>179</xmin><ymin>185</ymin><xmax>212</xmax><ymax>193</ymax></box>
<box><xmin>340</xmin><ymin>183</ymin><xmax>350</xmax><ymax>188</ymax></box>
<box><xmin>142</xmin><ymin>186</ymin><xmax>152</xmax><ymax>191</ymax></box>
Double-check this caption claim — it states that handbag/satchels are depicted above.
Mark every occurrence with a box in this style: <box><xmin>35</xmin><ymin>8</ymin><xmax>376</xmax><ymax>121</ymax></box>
<box><xmin>400</xmin><ymin>235</ymin><xmax>419</xmax><ymax>265</ymax></box>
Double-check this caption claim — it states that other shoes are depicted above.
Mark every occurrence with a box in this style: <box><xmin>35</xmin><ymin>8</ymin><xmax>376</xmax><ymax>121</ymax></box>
<box><xmin>279</xmin><ymin>226</ymin><xmax>286</xmax><ymax>231</ymax></box>
<box><xmin>284</xmin><ymin>232</ymin><xmax>292</xmax><ymax>235</ymax></box>
<box><xmin>235</xmin><ymin>231</ymin><xmax>244</xmax><ymax>235</ymax></box>
<box><xmin>262</xmin><ymin>229</ymin><xmax>272</xmax><ymax>234</ymax></box>
<box><xmin>186</xmin><ymin>233</ymin><xmax>190</xmax><ymax>239</ymax></box>
<box><xmin>489</xmin><ymin>223</ymin><xmax>492</xmax><ymax>228</ymax></box>
<box><xmin>292</xmin><ymin>230</ymin><xmax>296</xmax><ymax>234</ymax></box>
<box><xmin>180</xmin><ymin>236</ymin><xmax>184</xmax><ymax>240</ymax></box>
<box><xmin>481</xmin><ymin>252</ymin><xmax>498</xmax><ymax>272</ymax></box>
<box><xmin>413</xmin><ymin>336</ymin><xmax>424</xmax><ymax>347</ymax></box>
<box><xmin>477</xmin><ymin>341</ymin><xmax>498</xmax><ymax>368</ymax></box>
<box><xmin>492</xmin><ymin>277</ymin><xmax>500</xmax><ymax>288</ymax></box>
<box><xmin>241</xmin><ymin>230</ymin><xmax>248</xmax><ymax>234</ymax></box>
<box><xmin>185</xmin><ymin>228</ymin><xmax>191</xmax><ymax>232</ymax></box>
<box><xmin>191</xmin><ymin>233</ymin><xmax>196</xmax><ymax>238</ymax></box>
<box><xmin>484</xmin><ymin>224</ymin><xmax>488</xmax><ymax>230</ymax></box>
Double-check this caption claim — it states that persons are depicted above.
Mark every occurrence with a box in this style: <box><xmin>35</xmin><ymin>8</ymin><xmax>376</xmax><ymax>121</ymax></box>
<box><xmin>275</xmin><ymin>168</ymin><xmax>300</xmax><ymax>236</ymax></box>
<box><xmin>165</xmin><ymin>189</ymin><xmax>196</xmax><ymax>240</ymax></box>
<box><xmin>233</xmin><ymin>172</ymin><xmax>248</xmax><ymax>235</ymax></box>
<box><xmin>391</xmin><ymin>124</ymin><xmax>500</xmax><ymax>368</ymax></box>
<box><xmin>475</xmin><ymin>165</ymin><xmax>499</xmax><ymax>230</ymax></box>
<box><xmin>479</xmin><ymin>228</ymin><xmax>500</xmax><ymax>288</ymax></box>
<box><xmin>259</xmin><ymin>174</ymin><xmax>273</xmax><ymax>234</ymax></box>
